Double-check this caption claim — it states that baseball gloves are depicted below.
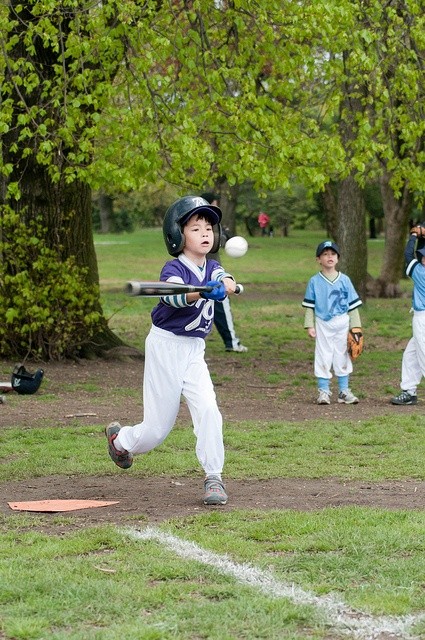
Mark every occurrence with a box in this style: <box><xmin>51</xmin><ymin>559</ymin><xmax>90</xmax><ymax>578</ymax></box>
<box><xmin>410</xmin><ymin>225</ymin><xmax>424</xmax><ymax>236</ymax></box>
<box><xmin>348</xmin><ymin>328</ymin><xmax>364</xmax><ymax>360</ymax></box>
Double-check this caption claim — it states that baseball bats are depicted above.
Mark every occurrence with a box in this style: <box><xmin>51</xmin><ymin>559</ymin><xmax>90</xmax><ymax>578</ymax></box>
<box><xmin>125</xmin><ymin>280</ymin><xmax>243</xmax><ymax>297</ymax></box>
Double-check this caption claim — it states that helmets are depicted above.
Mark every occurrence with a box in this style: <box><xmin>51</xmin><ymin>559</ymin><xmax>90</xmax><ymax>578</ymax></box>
<box><xmin>11</xmin><ymin>363</ymin><xmax>44</xmax><ymax>394</ymax></box>
<box><xmin>162</xmin><ymin>196</ymin><xmax>222</xmax><ymax>255</ymax></box>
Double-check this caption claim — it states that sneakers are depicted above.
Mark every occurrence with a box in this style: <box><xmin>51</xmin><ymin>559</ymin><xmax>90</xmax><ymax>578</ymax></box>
<box><xmin>104</xmin><ymin>422</ymin><xmax>133</xmax><ymax>469</ymax></box>
<box><xmin>337</xmin><ymin>389</ymin><xmax>360</xmax><ymax>404</ymax></box>
<box><xmin>317</xmin><ymin>389</ymin><xmax>332</xmax><ymax>404</ymax></box>
<box><xmin>225</xmin><ymin>344</ymin><xmax>248</xmax><ymax>352</ymax></box>
<box><xmin>203</xmin><ymin>474</ymin><xmax>228</xmax><ymax>504</ymax></box>
<box><xmin>390</xmin><ymin>391</ymin><xmax>418</xmax><ymax>405</ymax></box>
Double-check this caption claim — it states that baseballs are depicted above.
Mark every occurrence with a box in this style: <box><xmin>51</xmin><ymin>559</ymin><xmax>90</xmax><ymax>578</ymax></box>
<box><xmin>224</xmin><ymin>236</ymin><xmax>249</xmax><ymax>257</ymax></box>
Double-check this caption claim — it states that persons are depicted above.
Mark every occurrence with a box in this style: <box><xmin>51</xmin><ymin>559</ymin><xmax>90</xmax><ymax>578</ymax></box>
<box><xmin>302</xmin><ymin>240</ymin><xmax>364</xmax><ymax>405</ymax></box>
<box><xmin>390</xmin><ymin>227</ymin><xmax>425</xmax><ymax>406</ymax></box>
<box><xmin>105</xmin><ymin>196</ymin><xmax>235</xmax><ymax>505</ymax></box>
<box><xmin>257</xmin><ymin>210</ymin><xmax>272</xmax><ymax>237</ymax></box>
<box><xmin>198</xmin><ymin>193</ymin><xmax>249</xmax><ymax>353</ymax></box>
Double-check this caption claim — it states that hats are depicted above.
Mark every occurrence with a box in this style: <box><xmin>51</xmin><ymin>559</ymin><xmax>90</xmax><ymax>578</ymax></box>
<box><xmin>316</xmin><ymin>241</ymin><xmax>340</xmax><ymax>256</ymax></box>
